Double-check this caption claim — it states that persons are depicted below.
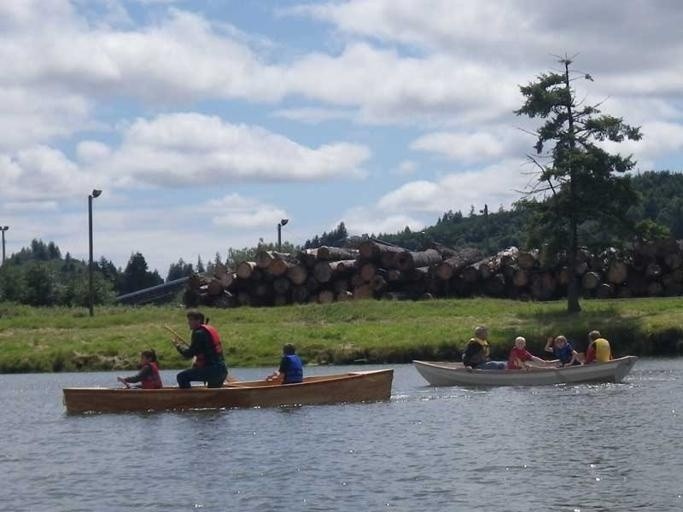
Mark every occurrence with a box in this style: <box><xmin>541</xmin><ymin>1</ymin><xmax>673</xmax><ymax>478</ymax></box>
<box><xmin>507</xmin><ymin>337</ymin><xmax>545</xmax><ymax>369</ymax></box>
<box><xmin>545</xmin><ymin>336</ymin><xmax>581</xmax><ymax>367</ymax></box>
<box><xmin>465</xmin><ymin>327</ymin><xmax>504</xmax><ymax>369</ymax></box>
<box><xmin>583</xmin><ymin>330</ymin><xmax>613</xmax><ymax>363</ymax></box>
<box><xmin>273</xmin><ymin>343</ymin><xmax>303</xmax><ymax>384</ymax></box>
<box><xmin>173</xmin><ymin>310</ymin><xmax>228</xmax><ymax>388</ymax></box>
<box><xmin>118</xmin><ymin>351</ymin><xmax>163</xmax><ymax>389</ymax></box>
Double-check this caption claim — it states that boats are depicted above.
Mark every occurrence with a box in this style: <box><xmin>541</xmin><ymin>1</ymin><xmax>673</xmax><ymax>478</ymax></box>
<box><xmin>408</xmin><ymin>355</ymin><xmax>640</xmax><ymax>389</ymax></box>
<box><xmin>61</xmin><ymin>368</ymin><xmax>394</xmax><ymax>415</ymax></box>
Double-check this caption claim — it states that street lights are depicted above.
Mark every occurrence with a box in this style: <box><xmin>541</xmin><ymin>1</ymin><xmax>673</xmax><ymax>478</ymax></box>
<box><xmin>276</xmin><ymin>218</ymin><xmax>289</xmax><ymax>251</ymax></box>
<box><xmin>87</xmin><ymin>187</ymin><xmax>102</xmax><ymax>316</ymax></box>
<box><xmin>0</xmin><ymin>224</ymin><xmax>11</xmax><ymax>265</ymax></box>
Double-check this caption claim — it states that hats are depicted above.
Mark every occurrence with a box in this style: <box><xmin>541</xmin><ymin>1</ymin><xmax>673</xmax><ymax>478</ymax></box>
<box><xmin>475</xmin><ymin>326</ymin><xmax>489</xmax><ymax>337</ymax></box>
